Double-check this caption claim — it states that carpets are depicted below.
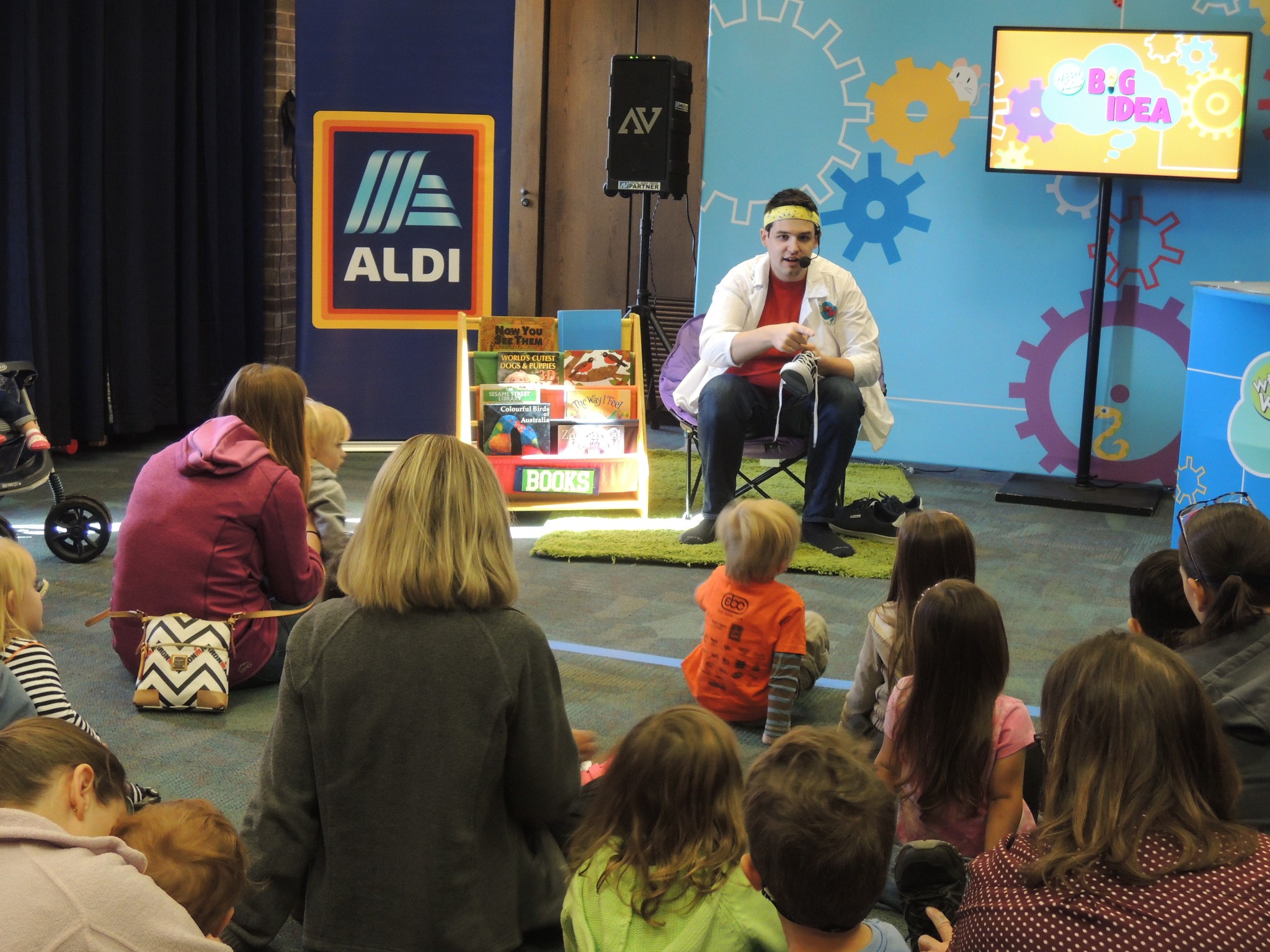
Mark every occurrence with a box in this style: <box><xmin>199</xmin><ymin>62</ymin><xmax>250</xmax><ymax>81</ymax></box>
<box><xmin>527</xmin><ymin>447</ymin><xmax>925</xmax><ymax>580</ymax></box>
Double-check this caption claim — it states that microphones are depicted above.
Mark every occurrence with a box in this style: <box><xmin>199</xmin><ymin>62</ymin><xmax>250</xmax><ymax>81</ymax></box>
<box><xmin>799</xmin><ymin>244</ymin><xmax>819</xmax><ymax>269</ymax></box>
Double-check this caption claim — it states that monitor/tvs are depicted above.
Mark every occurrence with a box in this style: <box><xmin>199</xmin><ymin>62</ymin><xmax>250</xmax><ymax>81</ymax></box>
<box><xmin>985</xmin><ymin>26</ymin><xmax>1253</xmax><ymax>183</ymax></box>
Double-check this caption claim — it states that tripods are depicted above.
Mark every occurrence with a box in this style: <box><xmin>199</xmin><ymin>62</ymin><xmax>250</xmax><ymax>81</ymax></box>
<box><xmin>623</xmin><ymin>192</ymin><xmax>672</xmax><ymax>431</ymax></box>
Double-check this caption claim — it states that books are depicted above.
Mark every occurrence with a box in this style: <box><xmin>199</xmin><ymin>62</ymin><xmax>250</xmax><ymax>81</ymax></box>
<box><xmin>473</xmin><ymin>309</ymin><xmax>638</xmax><ymax>457</ymax></box>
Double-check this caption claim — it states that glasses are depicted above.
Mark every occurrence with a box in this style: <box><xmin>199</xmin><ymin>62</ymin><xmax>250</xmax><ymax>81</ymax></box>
<box><xmin>1176</xmin><ymin>492</ymin><xmax>1259</xmax><ymax>602</ymax></box>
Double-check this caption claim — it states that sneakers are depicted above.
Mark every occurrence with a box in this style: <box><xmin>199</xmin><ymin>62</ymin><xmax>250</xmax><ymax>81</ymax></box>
<box><xmin>772</xmin><ymin>349</ymin><xmax>821</xmax><ymax>448</ymax></box>
<box><xmin>829</xmin><ymin>493</ymin><xmax>906</xmax><ymax>544</ymax></box>
<box><xmin>878</xmin><ymin>491</ymin><xmax>924</xmax><ymax>516</ymax></box>
<box><xmin>891</xmin><ymin>840</ymin><xmax>967</xmax><ymax>952</ymax></box>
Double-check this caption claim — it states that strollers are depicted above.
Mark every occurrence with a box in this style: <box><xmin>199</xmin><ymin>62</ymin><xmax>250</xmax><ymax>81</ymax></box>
<box><xmin>0</xmin><ymin>359</ymin><xmax>114</xmax><ymax>564</ymax></box>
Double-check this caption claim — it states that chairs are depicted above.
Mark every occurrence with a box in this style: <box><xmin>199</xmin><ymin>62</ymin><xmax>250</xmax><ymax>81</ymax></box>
<box><xmin>629</xmin><ymin>303</ymin><xmax>888</xmax><ymax>521</ymax></box>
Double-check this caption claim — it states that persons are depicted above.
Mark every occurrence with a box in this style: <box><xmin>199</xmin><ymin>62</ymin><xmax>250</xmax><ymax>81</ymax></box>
<box><xmin>0</xmin><ymin>535</ymin><xmax>252</xmax><ymax>952</ymax></box>
<box><xmin>739</xmin><ymin>725</ymin><xmax>968</xmax><ymax>952</ymax></box>
<box><xmin>872</xmin><ymin>577</ymin><xmax>1044</xmax><ymax>860</ymax></box>
<box><xmin>1172</xmin><ymin>501</ymin><xmax>1270</xmax><ymax>836</ymax></box>
<box><xmin>559</xmin><ymin>704</ymin><xmax>788</xmax><ymax>952</ymax></box>
<box><xmin>919</xmin><ymin>627</ymin><xmax>1270</xmax><ymax>952</ymax></box>
<box><xmin>218</xmin><ymin>431</ymin><xmax>600</xmax><ymax>952</ymax></box>
<box><xmin>837</xmin><ymin>508</ymin><xmax>976</xmax><ymax>759</ymax></box>
<box><xmin>1128</xmin><ymin>549</ymin><xmax>1200</xmax><ymax>652</ymax></box>
<box><xmin>304</xmin><ymin>396</ymin><xmax>352</xmax><ymax>601</ymax></box>
<box><xmin>108</xmin><ymin>362</ymin><xmax>326</xmax><ymax>690</ymax></box>
<box><xmin>681</xmin><ymin>498</ymin><xmax>832</xmax><ymax>746</ymax></box>
<box><xmin>0</xmin><ymin>392</ymin><xmax>51</xmax><ymax>452</ymax></box>
<box><xmin>671</xmin><ymin>187</ymin><xmax>895</xmax><ymax>559</ymax></box>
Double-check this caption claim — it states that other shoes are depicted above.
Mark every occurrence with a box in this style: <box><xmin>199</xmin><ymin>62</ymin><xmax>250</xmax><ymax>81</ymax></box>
<box><xmin>34</xmin><ymin>579</ymin><xmax>49</xmax><ymax>597</ymax></box>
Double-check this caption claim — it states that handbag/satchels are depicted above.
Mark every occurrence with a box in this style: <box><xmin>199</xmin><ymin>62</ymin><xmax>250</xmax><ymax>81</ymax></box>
<box><xmin>133</xmin><ymin>606</ymin><xmax>236</xmax><ymax>713</ymax></box>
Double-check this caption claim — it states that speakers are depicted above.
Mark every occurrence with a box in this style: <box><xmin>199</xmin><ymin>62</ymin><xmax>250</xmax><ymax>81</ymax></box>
<box><xmin>603</xmin><ymin>53</ymin><xmax>694</xmax><ymax>200</ymax></box>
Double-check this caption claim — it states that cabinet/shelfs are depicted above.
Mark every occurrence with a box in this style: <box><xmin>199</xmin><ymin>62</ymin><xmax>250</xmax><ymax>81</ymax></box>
<box><xmin>455</xmin><ymin>311</ymin><xmax>650</xmax><ymax>521</ymax></box>
